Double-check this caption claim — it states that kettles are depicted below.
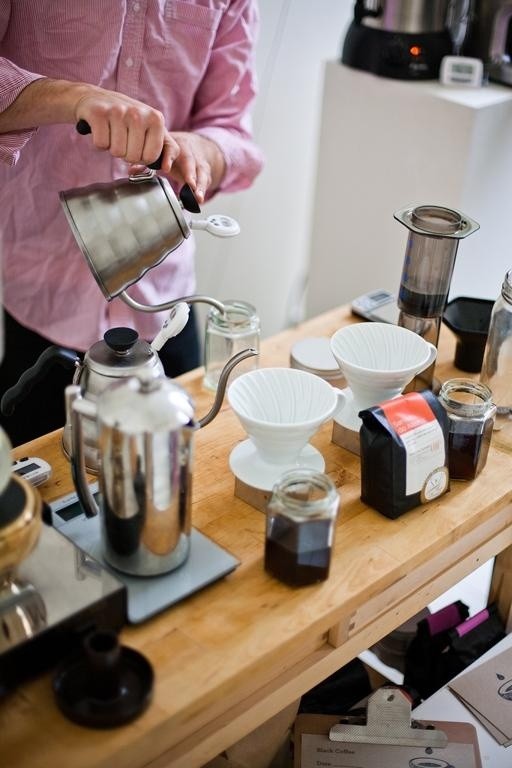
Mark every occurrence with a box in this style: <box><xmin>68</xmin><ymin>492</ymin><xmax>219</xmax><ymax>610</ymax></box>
<box><xmin>338</xmin><ymin>0</ymin><xmax>476</xmax><ymax>84</ymax></box>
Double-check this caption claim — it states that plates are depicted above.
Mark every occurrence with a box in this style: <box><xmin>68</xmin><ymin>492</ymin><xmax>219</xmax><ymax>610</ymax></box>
<box><xmin>287</xmin><ymin>333</ymin><xmax>349</xmax><ymax>384</ymax></box>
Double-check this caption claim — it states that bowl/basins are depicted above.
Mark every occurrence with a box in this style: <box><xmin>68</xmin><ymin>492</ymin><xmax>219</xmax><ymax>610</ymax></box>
<box><xmin>438</xmin><ymin>289</ymin><xmax>512</xmax><ymax>376</ymax></box>
<box><xmin>324</xmin><ymin>317</ymin><xmax>444</xmax><ymax>436</ymax></box>
<box><xmin>224</xmin><ymin>359</ymin><xmax>349</xmax><ymax>498</ymax></box>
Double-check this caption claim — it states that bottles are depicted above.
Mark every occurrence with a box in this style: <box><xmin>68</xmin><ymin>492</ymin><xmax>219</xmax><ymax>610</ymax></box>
<box><xmin>432</xmin><ymin>374</ymin><xmax>500</xmax><ymax>486</ymax></box>
<box><xmin>473</xmin><ymin>260</ymin><xmax>512</xmax><ymax>419</ymax></box>
<box><xmin>259</xmin><ymin>467</ymin><xmax>339</xmax><ymax>588</ymax></box>
<box><xmin>390</xmin><ymin>192</ymin><xmax>482</xmax><ymax>394</ymax></box>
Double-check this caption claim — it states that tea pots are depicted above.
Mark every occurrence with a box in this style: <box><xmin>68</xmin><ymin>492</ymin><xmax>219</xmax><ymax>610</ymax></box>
<box><xmin>23</xmin><ymin>112</ymin><xmax>273</xmax><ymax>327</ymax></box>
<box><xmin>68</xmin><ymin>347</ymin><xmax>260</xmax><ymax>581</ymax></box>
<box><xmin>60</xmin><ymin>300</ymin><xmax>190</xmax><ymax>477</ymax></box>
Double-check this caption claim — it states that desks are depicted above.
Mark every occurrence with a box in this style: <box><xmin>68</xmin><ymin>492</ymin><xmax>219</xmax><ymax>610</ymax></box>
<box><xmin>0</xmin><ymin>294</ymin><xmax>512</xmax><ymax>767</ymax></box>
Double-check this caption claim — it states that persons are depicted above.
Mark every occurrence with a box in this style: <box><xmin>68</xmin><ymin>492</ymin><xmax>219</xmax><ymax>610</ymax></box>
<box><xmin>0</xmin><ymin>0</ymin><xmax>262</xmax><ymax>450</ymax></box>
